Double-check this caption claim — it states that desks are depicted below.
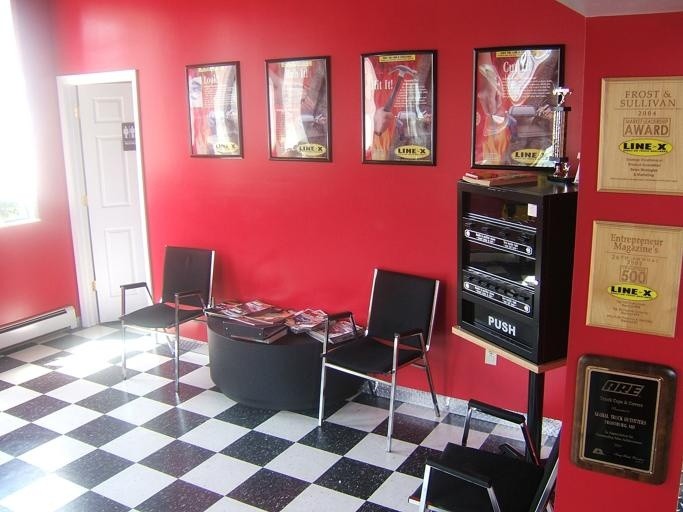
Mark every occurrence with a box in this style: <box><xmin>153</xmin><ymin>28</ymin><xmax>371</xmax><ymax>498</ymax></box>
<box><xmin>207</xmin><ymin>300</ymin><xmax>365</xmax><ymax>411</ymax></box>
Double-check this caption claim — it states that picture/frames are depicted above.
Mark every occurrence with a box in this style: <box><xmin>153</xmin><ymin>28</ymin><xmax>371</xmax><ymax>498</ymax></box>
<box><xmin>471</xmin><ymin>43</ymin><xmax>566</xmax><ymax>171</ymax></box>
<box><xmin>596</xmin><ymin>76</ymin><xmax>683</xmax><ymax>196</ymax></box>
<box><xmin>360</xmin><ymin>49</ymin><xmax>437</xmax><ymax>167</ymax></box>
<box><xmin>184</xmin><ymin>61</ymin><xmax>244</xmax><ymax>159</ymax></box>
<box><xmin>264</xmin><ymin>55</ymin><xmax>331</xmax><ymax>162</ymax></box>
<box><xmin>585</xmin><ymin>220</ymin><xmax>683</xmax><ymax>338</ymax></box>
<box><xmin>570</xmin><ymin>355</ymin><xmax>676</xmax><ymax>486</ymax></box>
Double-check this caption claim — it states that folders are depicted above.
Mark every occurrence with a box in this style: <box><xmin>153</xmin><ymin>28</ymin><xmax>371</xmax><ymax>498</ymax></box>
<box><xmin>223</xmin><ymin>319</ymin><xmax>286</xmax><ymax>340</ymax></box>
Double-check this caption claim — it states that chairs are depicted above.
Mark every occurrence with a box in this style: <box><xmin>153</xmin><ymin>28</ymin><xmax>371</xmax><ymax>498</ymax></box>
<box><xmin>319</xmin><ymin>268</ymin><xmax>441</xmax><ymax>453</ymax></box>
<box><xmin>407</xmin><ymin>399</ymin><xmax>561</xmax><ymax>512</ymax></box>
<box><xmin>118</xmin><ymin>245</ymin><xmax>216</xmax><ymax>394</ymax></box>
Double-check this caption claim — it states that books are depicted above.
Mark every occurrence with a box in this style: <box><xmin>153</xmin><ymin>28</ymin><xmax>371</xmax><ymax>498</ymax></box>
<box><xmin>203</xmin><ymin>298</ymin><xmax>365</xmax><ymax>345</ymax></box>
<box><xmin>462</xmin><ymin>171</ymin><xmax>537</xmax><ymax>186</ymax></box>
